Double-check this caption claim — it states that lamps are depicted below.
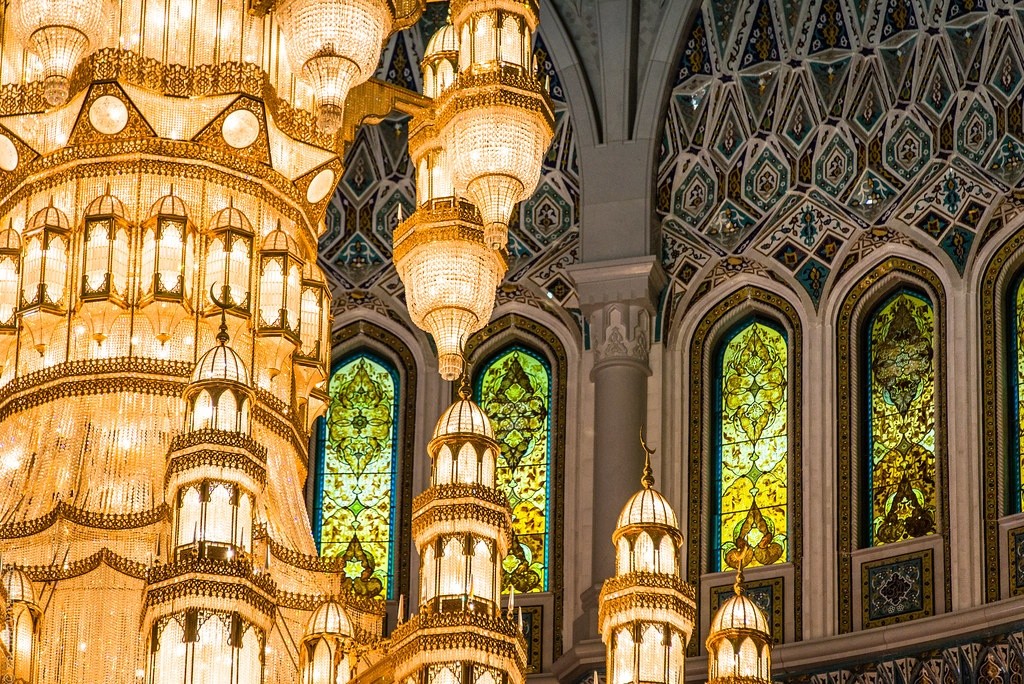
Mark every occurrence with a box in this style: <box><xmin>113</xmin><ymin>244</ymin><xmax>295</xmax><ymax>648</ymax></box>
<box><xmin>1</xmin><ymin>2</ymin><xmax>775</xmax><ymax>682</ymax></box>
